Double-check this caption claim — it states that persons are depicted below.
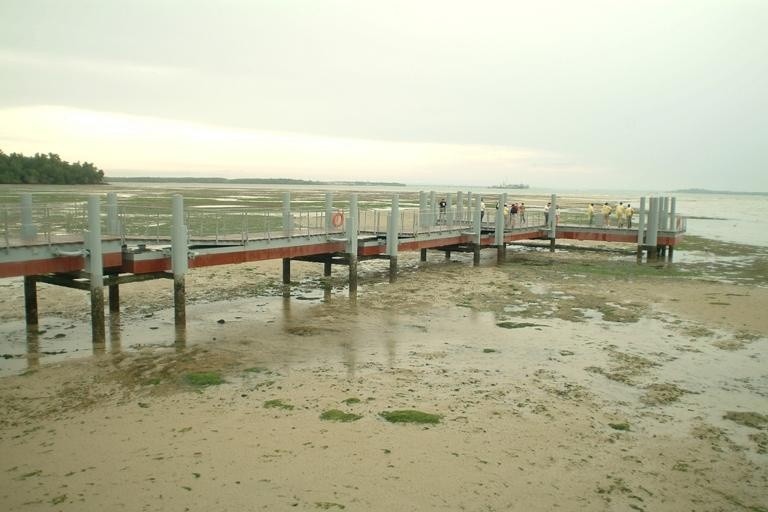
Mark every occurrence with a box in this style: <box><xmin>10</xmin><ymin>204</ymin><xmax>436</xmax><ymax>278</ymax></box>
<box><xmin>480</xmin><ymin>198</ymin><xmax>635</xmax><ymax>230</ymax></box>
<box><xmin>438</xmin><ymin>199</ymin><xmax>448</xmax><ymax>222</ymax></box>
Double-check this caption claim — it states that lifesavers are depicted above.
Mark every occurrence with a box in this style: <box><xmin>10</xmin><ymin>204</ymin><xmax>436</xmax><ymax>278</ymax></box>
<box><xmin>332</xmin><ymin>213</ymin><xmax>343</xmax><ymax>227</ymax></box>
